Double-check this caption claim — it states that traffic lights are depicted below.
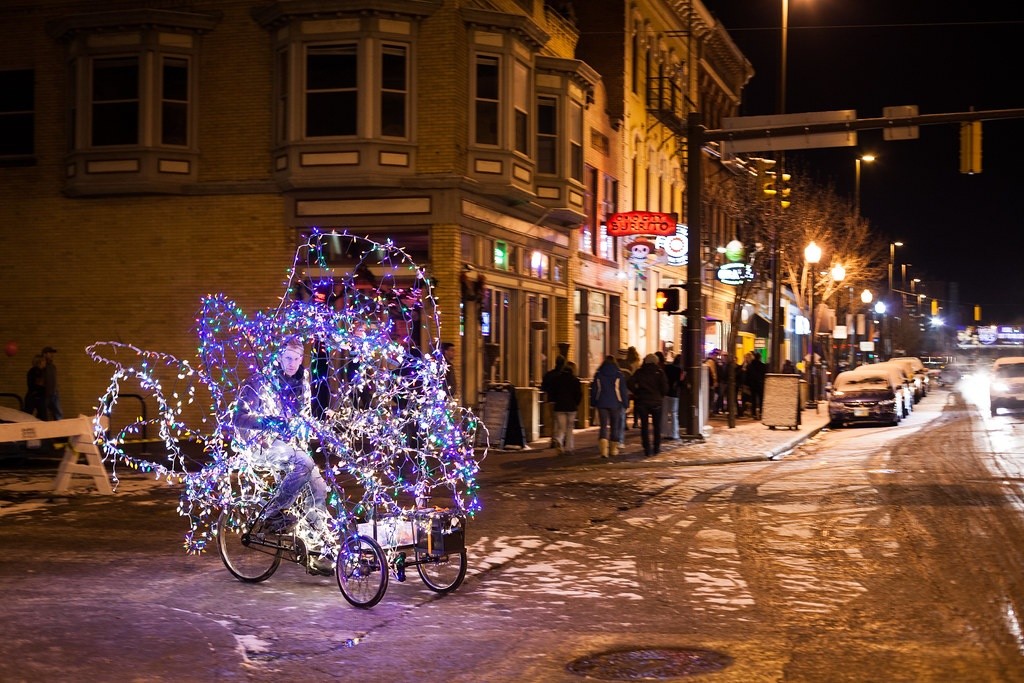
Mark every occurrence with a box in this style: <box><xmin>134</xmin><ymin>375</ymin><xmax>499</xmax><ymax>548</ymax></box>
<box><xmin>655</xmin><ymin>287</ymin><xmax>680</xmax><ymax>312</ymax></box>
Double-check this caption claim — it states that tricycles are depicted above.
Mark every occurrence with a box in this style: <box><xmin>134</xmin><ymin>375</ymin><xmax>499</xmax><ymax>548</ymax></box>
<box><xmin>217</xmin><ymin>467</ymin><xmax>468</xmax><ymax>610</ymax></box>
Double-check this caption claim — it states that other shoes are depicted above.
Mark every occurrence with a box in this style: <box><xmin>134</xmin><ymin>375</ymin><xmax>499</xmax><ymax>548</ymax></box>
<box><xmin>646</xmin><ymin>450</ymin><xmax>651</xmax><ymax>456</ymax></box>
<box><xmin>263</xmin><ymin>510</ymin><xmax>292</xmax><ymax>532</ymax></box>
<box><xmin>619</xmin><ymin>444</ymin><xmax>626</xmax><ymax>449</ymax></box>
<box><xmin>633</xmin><ymin>423</ymin><xmax>639</xmax><ymax>428</ymax></box>
<box><xmin>713</xmin><ymin>412</ymin><xmax>723</xmax><ymax>416</ymax></box>
<box><xmin>309</xmin><ymin>553</ymin><xmax>334</xmax><ymax>575</ymax></box>
<box><xmin>654</xmin><ymin>448</ymin><xmax>663</xmax><ymax>453</ymax></box>
<box><xmin>554</xmin><ymin>438</ymin><xmax>564</xmax><ymax>453</ymax></box>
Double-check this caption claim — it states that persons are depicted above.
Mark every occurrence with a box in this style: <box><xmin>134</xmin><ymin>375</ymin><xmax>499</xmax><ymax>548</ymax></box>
<box><xmin>23</xmin><ymin>356</ymin><xmax>52</xmax><ymax>457</ymax></box>
<box><xmin>553</xmin><ymin>361</ymin><xmax>582</xmax><ymax>455</ymax></box>
<box><xmin>41</xmin><ymin>346</ymin><xmax>66</xmax><ymax>443</ymax></box>
<box><xmin>623</xmin><ymin>346</ymin><xmax>793</xmax><ymax>439</ymax></box>
<box><xmin>588</xmin><ymin>355</ymin><xmax>628</xmax><ymax>458</ymax></box>
<box><xmin>442</xmin><ymin>341</ymin><xmax>458</xmax><ymax>396</ymax></box>
<box><xmin>232</xmin><ymin>339</ymin><xmax>336</xmax><ymax>574</ymax></box>
<box><xmin>627</xmin><ymin>354</ymin><xmax>667</xmax><ymax>456</ymax></box>
<box><xmin>542</xmin><ymin>355</ymin><xmax>566</xmax><ymax>445</ymax></box>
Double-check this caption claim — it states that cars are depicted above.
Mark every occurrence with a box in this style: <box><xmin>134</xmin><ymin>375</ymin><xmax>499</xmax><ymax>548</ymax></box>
<box><xmin>990</xmin><ymin>356</ymin><xmax>1024</xmax><ymax>418</ymax></box>
<box><xmin>937</xmin><ymin>364</ymin><xmax>979</xmax><ymax>387</ymax></box>
<box><xmin>853</xmin><ymin>356</ymin><xmax>930</xmax><ymax>419</ymax></box>
<box><xmin>824</xmin><ymin>369</ymin><xmax>904</xmax><ymax>429</ymax></box>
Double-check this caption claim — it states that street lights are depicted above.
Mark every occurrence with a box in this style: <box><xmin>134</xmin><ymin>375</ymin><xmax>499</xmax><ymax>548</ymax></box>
<box><xmin>901</xmin><ymin>263</ymin><xmax>913</xmax><ymax>306</ymax></box>
<box><xmin>911</xmin><ymin>278</ymin><xmax>922</xmax><ymax>303</ymax></box>
<box><xmin>888</xmin><ymin>241</ymin><xmax>903</xmax><ymax>310</ymax></box>
<box><xmin>831</xmin><ymin>262</ymin><xmax>846</xmax><ymax>374</ymax></box>
<box><xmin>860</xmin><ymin>288</ymin><xmax>872</xmax><ymax>363</ymax></box>
<box><xmin>854</xmin><ymin>155</ymin><xmax>875</xmax><ymax>220</ymax></box>
<box><xmin>875</xmin><ymin>301</ymin><xmax>886</xmax><ymax>363</ymax></box>
<box><xmin>916</xmin><ymin>294</ymin><xmax>927</xmax><ymax>312</ymax></box>
<box><xmin>804</xmin><ymin>239</ymin><xmax>822</xmax><ymax>408</ymax></box>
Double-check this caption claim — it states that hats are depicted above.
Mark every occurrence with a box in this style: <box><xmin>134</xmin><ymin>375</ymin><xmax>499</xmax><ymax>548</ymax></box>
<box><xmin>279</xmin><ymin>334</ymin><xmax>305</xmax><ymax>350</ymax></box>
<box><xmin>42</xmin><ymin>347</ymin><xmax>57</xmax><ymax>353</ymax></box>
<box><xmin>750</xmin><ymin>350</ymin><xmax>761</xmax><ymax>360</ymax></box>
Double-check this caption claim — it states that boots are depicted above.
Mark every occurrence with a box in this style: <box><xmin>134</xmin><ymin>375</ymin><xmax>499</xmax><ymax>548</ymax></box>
<box><xmin>610</xmin><ymin>441</ymin><xmax>619</xmax><ymax>456</ymax></box>
<box><xmin>599</xmin><ymin>439</ymin><xmax>609</xmax><ymax>458</ymax></box>
